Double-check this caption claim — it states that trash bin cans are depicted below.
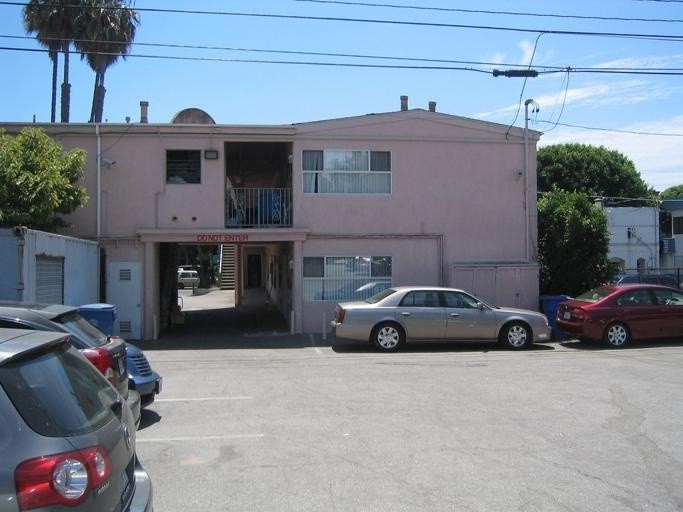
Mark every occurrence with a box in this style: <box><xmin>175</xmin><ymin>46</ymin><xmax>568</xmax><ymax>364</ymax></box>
<box><xmin>79</xmin><ymin>303</ymin><xmax>120</xmax><ymax>335</ymax></box>
<box><xmin>540</xmin><ymin>295</ymin><xmax>571</xmax><ymax>341</ymax></box>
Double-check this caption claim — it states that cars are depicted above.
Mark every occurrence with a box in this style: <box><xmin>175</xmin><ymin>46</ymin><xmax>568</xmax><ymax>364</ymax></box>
<box><xmin>554</xmin><ymin>273</ymin><xmax>683</xmax><ymax>349</ymax></box>
<box><xmin>331</xmin><ymin>287</ymin><xmax>552</xmax><ymax>353</ymax></box>
<box><xmin>177</xmin><ymin>270</ymin><xmax>200</xmax><ymax>289</ymax></box>
<box><xmin>0</xmin><ymin>300</ymin><xmax>163</xmax><ymax>512</ymax></box>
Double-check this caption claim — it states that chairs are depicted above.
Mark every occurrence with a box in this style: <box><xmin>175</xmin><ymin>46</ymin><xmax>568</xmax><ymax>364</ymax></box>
<box><xmin>424</xmin><ymin>292</ymin><xmax>434</xmax><ymax>307</ymax></box>
